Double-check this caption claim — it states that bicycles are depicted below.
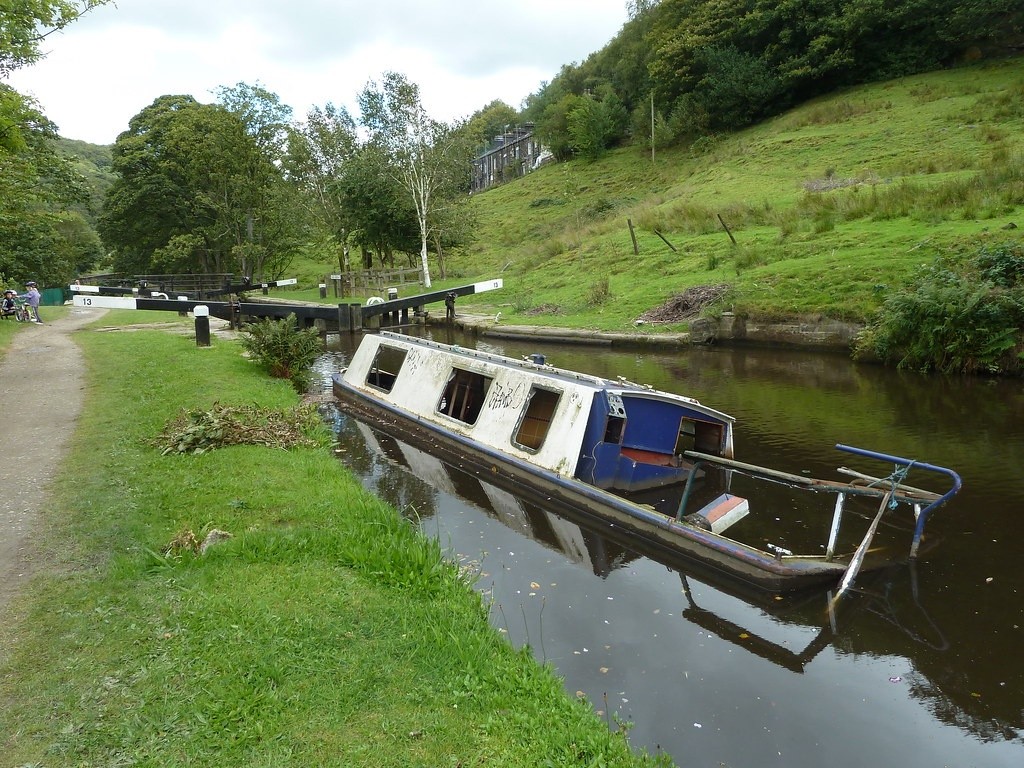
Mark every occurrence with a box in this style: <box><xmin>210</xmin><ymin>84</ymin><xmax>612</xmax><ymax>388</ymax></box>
<box><xmin>14</xmin><ymin>297</ymin><xmax>32</xmax><ymax>324</ymax></box>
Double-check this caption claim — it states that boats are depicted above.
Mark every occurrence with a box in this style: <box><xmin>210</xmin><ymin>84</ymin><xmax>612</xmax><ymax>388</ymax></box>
<box><xmin>332</xmin><ymin>330</ymin><xmax>964</xmax><ymax>597</ymax></box>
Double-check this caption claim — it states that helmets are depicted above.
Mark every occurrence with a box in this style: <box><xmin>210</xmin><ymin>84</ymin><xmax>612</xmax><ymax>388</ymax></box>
<box><xmin>30</xmin><ymin>282</ymin><xmax>36</xmax><ymax>286</ymax></box>
<box><xmin>25</xmin><ymin>282</ymin><xmax>31</xmax><ymax>286</ymax></box>
<box><xmin>4</xmin><ymin>291</ymin><xmax>12</xmax><ymax>294</ymax></box>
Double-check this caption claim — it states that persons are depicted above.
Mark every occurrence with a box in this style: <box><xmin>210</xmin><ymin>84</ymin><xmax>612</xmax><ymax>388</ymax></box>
<box><xmin>16</xmin><ymin>281</ymin><xmax>43</xmax><ymax>324</ymax></box>
<box><xmin>2</xmin><ymin>290</ymin><xmax>22</xmax><ymax>322</ymax></box>
